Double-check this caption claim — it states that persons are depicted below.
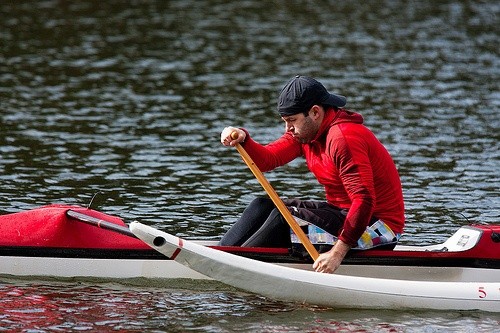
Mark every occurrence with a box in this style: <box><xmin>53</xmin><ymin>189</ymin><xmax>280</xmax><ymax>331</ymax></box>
<box><xmin>215</xmin><ymin>75</ymin><xmax>404</xmax><ymax>274</ymax></box>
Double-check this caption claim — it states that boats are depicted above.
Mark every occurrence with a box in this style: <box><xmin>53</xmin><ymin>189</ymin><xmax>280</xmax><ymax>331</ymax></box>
<box><xmin>0</xmin><ymin>193</ymin><xmax>500</xmax><ymax>277</ymax></box>
<box><xmin>128</xmin><ymin>221</ymin><xmax>500</xmax><ymax>313</ymax></box>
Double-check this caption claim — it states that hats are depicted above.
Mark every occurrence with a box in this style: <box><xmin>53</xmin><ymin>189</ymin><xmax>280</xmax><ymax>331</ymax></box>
<box><xmin>278</xmin><ymin>75</ymin><xmax>347</xmax><ymax>114</ymax></box>
